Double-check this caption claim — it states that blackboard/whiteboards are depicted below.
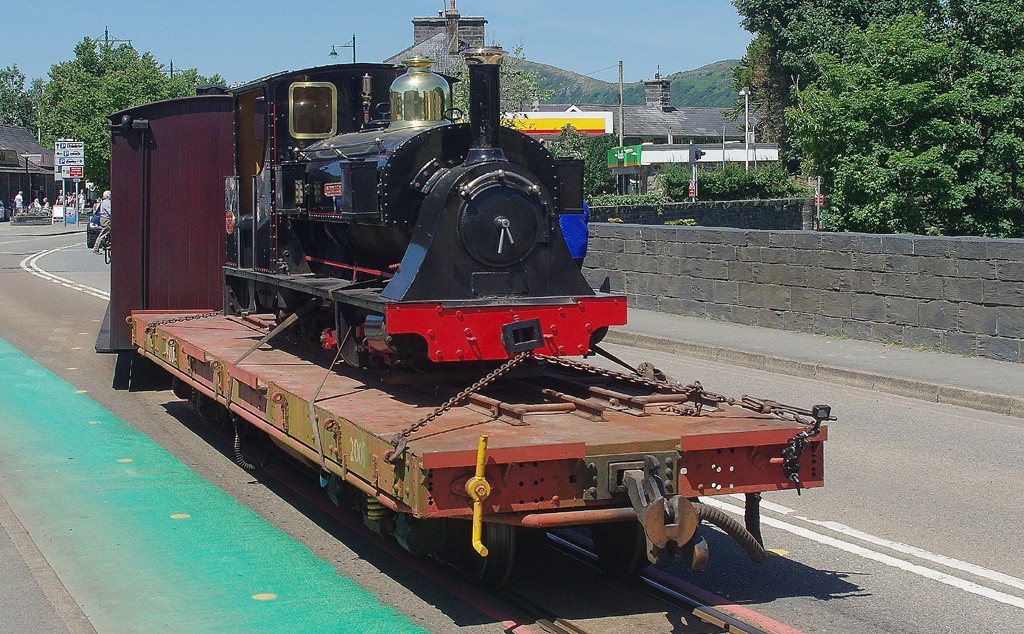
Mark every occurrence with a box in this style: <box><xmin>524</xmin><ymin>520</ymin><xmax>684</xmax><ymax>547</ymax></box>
<box><xmin>52</xmin><ymin>205</ymin><xmax>63</xmax><ymax>217</ymax></box>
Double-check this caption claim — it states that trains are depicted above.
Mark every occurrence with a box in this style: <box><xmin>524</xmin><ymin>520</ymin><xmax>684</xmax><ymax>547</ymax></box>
<box><xmin>90</xmin><ymin>1</ymin><xmax>840</xmax><ymax>600</ymax></box>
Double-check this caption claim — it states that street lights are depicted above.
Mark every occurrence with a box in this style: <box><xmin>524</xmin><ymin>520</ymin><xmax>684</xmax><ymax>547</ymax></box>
<box><xmin>328</xmin><ymin>32</ymin><xmax>356</xmax><ymax>63</ymax></box>
<box><xmin>738</xmin><ymin>87</ymin><xmax>751</xmax><ymax>179</ymax></box>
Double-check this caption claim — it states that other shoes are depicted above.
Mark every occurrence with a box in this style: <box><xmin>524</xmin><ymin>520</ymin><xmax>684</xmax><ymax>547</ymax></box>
<box><xmin>92</xmin><ymin>249</ymin><xmax>102</xmax><ymax>255</ymax></box>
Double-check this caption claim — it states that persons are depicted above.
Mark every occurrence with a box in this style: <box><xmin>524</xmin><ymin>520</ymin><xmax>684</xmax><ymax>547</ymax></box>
<box><xmin>93</xmin><ymin>190</ymin><xmax>111</xmax><ymax>255</ymax></box>
<box><xmin>56</xmin><ymin>188</ymin><xmax>86</xmax><ymax>213</ymax></box>
<box><xmin>0</xmin><ymin>191</ymin><xmax>49</xmax><ymax>216</ymax></box>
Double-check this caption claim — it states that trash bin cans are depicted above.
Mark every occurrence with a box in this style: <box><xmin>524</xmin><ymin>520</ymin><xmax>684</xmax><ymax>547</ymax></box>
<box><xmin>0</xmin><ymin>205</ymin><xmax>11</xmax><ymax>222</ymax></box>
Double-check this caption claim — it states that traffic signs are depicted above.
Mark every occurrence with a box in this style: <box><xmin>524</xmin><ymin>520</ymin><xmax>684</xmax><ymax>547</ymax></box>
<box><xmin>54</xmin><ymin>141</ymin><xmax>85</xmax><ymax>166</ymax></box>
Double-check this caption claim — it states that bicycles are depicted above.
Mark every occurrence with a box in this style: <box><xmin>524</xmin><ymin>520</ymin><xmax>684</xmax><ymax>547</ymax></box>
<box><xmin>98</xmin><ymin>225</ymin><xmax>112</xmax><ymax>264</ymax></box>
